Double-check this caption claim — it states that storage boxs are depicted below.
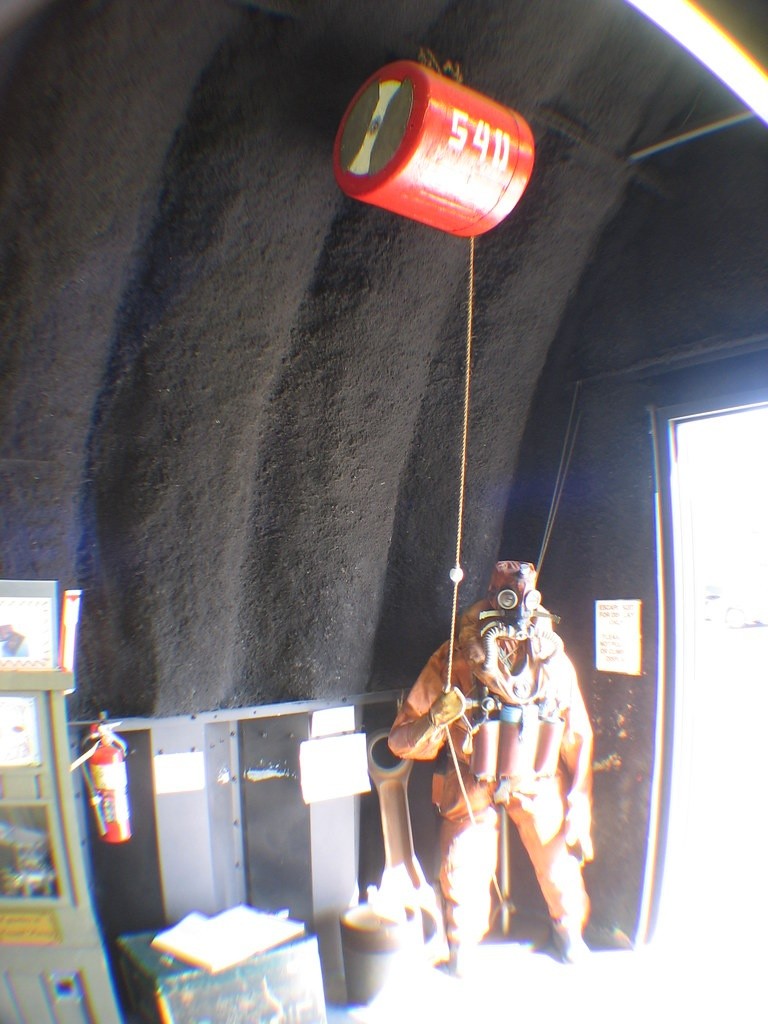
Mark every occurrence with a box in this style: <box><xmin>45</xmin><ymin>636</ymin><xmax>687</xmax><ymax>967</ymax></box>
<box><xmin>117</xmin><ymin>921</ymin><xmax>328</xmax><ymax>1024</ymax></box>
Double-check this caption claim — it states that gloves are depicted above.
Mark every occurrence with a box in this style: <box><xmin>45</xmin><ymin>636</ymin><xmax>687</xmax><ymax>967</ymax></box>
<box><xmin>565</xmin><ymin>792</ymin><xmax>598</xmax><ymax>862</ymax></box>
<box><xmin>428</xmin><ymin>685</ymin><xmax>467</xmax><ymax>727</ymax></box>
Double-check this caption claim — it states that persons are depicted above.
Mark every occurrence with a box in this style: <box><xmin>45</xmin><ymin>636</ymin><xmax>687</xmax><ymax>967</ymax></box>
<box><xmin>388</xmin><ymin>603</ymin><xmax>595</xmax><ymax>975</ymax></box>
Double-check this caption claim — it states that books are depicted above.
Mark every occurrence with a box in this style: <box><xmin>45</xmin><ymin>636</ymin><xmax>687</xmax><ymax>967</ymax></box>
<box><xmin>150</xmin><ymin>903</ymin><xmax>306</xmax><ymax>976</ymax></box>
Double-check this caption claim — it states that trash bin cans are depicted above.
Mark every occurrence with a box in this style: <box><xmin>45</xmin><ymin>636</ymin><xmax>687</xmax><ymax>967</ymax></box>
<box><xmin>342</xmin><ymin>899</ymin><xmax>425</xmax><ymax>1003</ymax></box>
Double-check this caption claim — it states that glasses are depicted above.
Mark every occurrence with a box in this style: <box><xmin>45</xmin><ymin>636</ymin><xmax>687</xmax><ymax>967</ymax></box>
<box><xmin>498</xmin><ymin>590</ymin><xmax>542</xmax><ymax>610</ymax></box>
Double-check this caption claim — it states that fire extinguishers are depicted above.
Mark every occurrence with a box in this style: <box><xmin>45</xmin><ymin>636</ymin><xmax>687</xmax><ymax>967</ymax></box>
<box><xmin>79</xmin><ymin>721</ymin><xmax>131</xmax><ymax>843</ymax></box>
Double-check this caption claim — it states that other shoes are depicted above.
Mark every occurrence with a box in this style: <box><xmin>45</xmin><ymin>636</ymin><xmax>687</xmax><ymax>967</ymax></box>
<box><xmin>450</xmin><ymin>949</ymin><xmax>476</xmax><ymax>980</ymax></box>
<box><xmin>553</xmin><ymin>928</ymin><xmax>590</xmax><ymax>966</ymax></box>
